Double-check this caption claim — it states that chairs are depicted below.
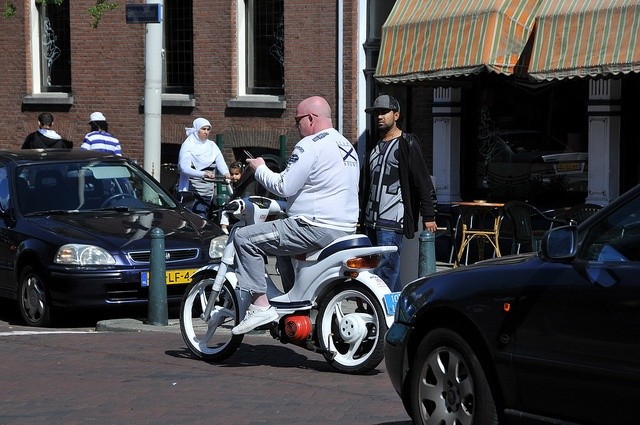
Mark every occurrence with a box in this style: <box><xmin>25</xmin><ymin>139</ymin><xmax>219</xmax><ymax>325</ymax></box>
<box><xmin>506</xmin><ymin>203</ymin><xmax>568</xmax><ymax>255</ymax></box>
<box><xmin>565</xmin><ymin>204</ymin><xmax>604</xmax><ymax>225</ymax></box>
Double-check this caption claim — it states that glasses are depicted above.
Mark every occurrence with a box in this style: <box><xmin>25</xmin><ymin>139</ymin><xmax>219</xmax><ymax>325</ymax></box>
<box><xmin>295</xmin><ymin>114</ymin><xmax>318</xmax><ymax>124</ymax></box>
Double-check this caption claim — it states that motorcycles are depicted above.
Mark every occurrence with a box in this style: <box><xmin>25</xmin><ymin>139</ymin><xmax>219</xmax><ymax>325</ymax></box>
<box><xmin>180</xmin><ymin>195</ymin><xmax>400</xmax><ymax>374</ymax></box>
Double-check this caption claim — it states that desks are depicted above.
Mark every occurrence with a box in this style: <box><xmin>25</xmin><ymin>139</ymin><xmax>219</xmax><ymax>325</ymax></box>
<box><xmin>450</xmin><ymin>198</ymin><xmax>506</xmax><ymax>269</ymax></box>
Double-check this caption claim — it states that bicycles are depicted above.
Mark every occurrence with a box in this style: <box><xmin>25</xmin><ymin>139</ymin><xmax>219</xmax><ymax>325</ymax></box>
<box><xmin>170</xmin><ymin>174</ymin><xmax>238</xmax><ymax>226</ymax></box>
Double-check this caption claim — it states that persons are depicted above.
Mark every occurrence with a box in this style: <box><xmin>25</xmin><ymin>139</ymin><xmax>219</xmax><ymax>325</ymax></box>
<box><xmin>232</xmin><ymin>96</ymin><xmax>361</xmax><ymax>334</ymax></box>
<box><xmin>21</xmin><ymin>113</ymin><xmax>65</xmax><ymax>149</ymax></box>
<box><xmin>80</xmin><ymin>112</ymin><xmax>122</xmax><ymax>156</ymax></box>
<box><xmin>229</xmin><ymin>162</ymin><xmax>245</xmax><ymax>184</ymax></box>
<box><xmin>177</xmin><ymin>118</ymin><xmax>231</xmax><ymax>219</ymax></box>
<box><xmin>363</xmin><ymin>95</ymin><xmax>437</xmax><ymax>293</ymax></box>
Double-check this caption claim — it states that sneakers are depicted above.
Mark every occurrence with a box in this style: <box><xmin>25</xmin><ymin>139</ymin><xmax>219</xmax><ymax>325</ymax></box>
<box><xmin>231</xmin><ymin>304</ymin><xmax>279</xmax><ymax>335</ymax></box>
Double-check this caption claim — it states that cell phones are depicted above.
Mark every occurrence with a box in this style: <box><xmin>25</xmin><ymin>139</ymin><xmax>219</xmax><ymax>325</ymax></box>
<box><xmin>242</xmin><ymin>150</ymin><xmax>255</xmax><ymax>159</ymax></box>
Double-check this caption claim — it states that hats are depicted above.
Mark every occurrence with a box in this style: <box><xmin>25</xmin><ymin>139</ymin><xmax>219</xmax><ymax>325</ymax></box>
<box><xmin>365</xmin><ymin>95</ymin><xmax>401</xmax><ymax>114</ymax></box>
<box><xmin>88</xmin><ymin>112</ymin><xmax>106</xmax><ymax>124</ymax></box>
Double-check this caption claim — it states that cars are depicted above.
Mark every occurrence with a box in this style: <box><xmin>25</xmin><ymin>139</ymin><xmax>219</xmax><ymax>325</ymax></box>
<box><xmin>0</xmin><ymin>149</ymin><xmax>229</xmax><ymax>327</ymax></box>
<box><xmin>385</xmin><ymin>184</ymin><xmax>639</xmax><ymax>425</ymax></box>
